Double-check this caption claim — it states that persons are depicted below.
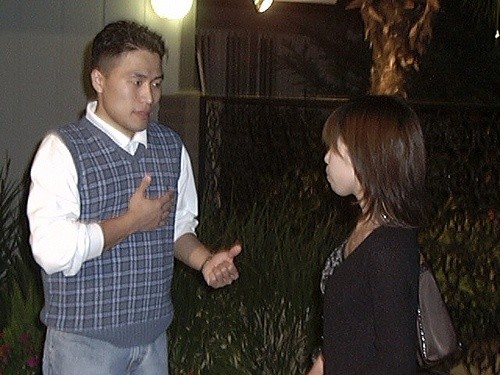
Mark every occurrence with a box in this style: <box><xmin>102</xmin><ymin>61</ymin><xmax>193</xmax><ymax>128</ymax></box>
<box><xmin>304</xmin><ymin>92</ymin><xmax>427</xmax><ymax>375</ymax></box>
<box><xmin>27</xmin><ymin>18</ymin><xmax>241</xmax><ymax>375</ymax></box>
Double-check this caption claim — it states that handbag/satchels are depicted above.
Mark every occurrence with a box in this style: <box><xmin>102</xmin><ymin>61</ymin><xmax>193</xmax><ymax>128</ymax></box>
<box><xmin>416</xmin><ymin>254</ymin><xmax>458</xmax><ymax>369</ymax></box>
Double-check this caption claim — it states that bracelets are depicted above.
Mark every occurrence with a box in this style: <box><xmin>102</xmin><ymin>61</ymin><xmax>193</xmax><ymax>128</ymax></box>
<box><xmin>312</xmin><ymin>347</ymin><xmax>322</xmax><ymax>360</ymax></box>
<box><xmin>199</xmin><ymin>254</ymin><xmax>213</xmax><ymax>272</ymax></box>
